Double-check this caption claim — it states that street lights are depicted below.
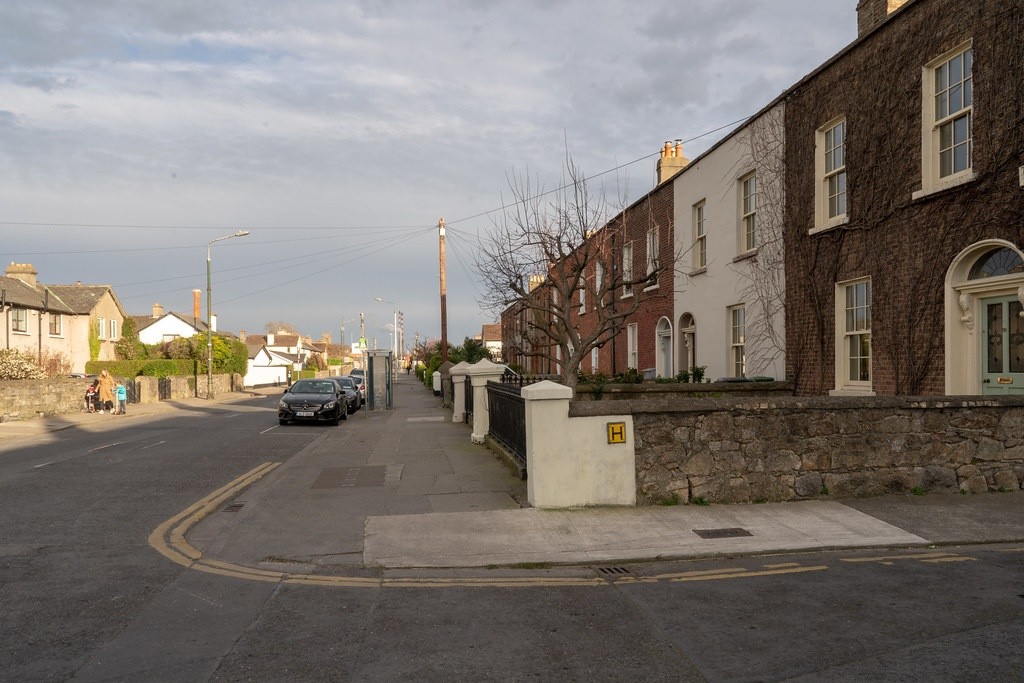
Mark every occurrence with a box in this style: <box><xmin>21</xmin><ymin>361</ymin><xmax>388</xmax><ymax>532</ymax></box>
<box><xmin>376</xmin><ymin>298</ymin><xmax>398</xmax><ymax>382</ymax></box>
<box><xmin>206</xmin><ymin>231</ymin><xmax>251</xmax><ymax>400</ymax></box>
<box><xmin>340</xmin><ymin>319</ymin><xmax>354</xmax><ymax>376</ymax></box>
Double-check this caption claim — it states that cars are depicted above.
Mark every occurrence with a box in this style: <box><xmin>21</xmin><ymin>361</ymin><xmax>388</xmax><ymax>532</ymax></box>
<box><xmin>328</xmin><ymin>369</ymin><xmax>368</xmax><ymax>415</ymax></box>
<box><xmin>278</xmin><ymin>378</ymin><xmax>348</xmax><ymax>427</ymax></box>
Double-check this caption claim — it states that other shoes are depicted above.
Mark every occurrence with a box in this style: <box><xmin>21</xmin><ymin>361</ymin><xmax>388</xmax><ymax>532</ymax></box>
<box><xmin>123</xmin><ymin>411</ymin><xmax>126</xmax><ymax>414</ymax></box>
<box><xmin>110</xmin><ymin>408</ymin><xmax>115</xmax><ymax>413</ymax></box>
<box><xmin>99</xmin><ymin>410</ymin><xmax>104</xmax><ymax>414</ymax></box>
<box><xmin>116</xmin><ymin>411</ymin><xmax>121</xmax><ymax>415</ymax></box>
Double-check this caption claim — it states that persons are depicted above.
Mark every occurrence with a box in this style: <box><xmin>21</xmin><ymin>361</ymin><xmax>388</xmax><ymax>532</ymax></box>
<box><xmin>85</xmin><ymin>370</ymin><xmax>126</xmax><ymax>415</ymax></box>
